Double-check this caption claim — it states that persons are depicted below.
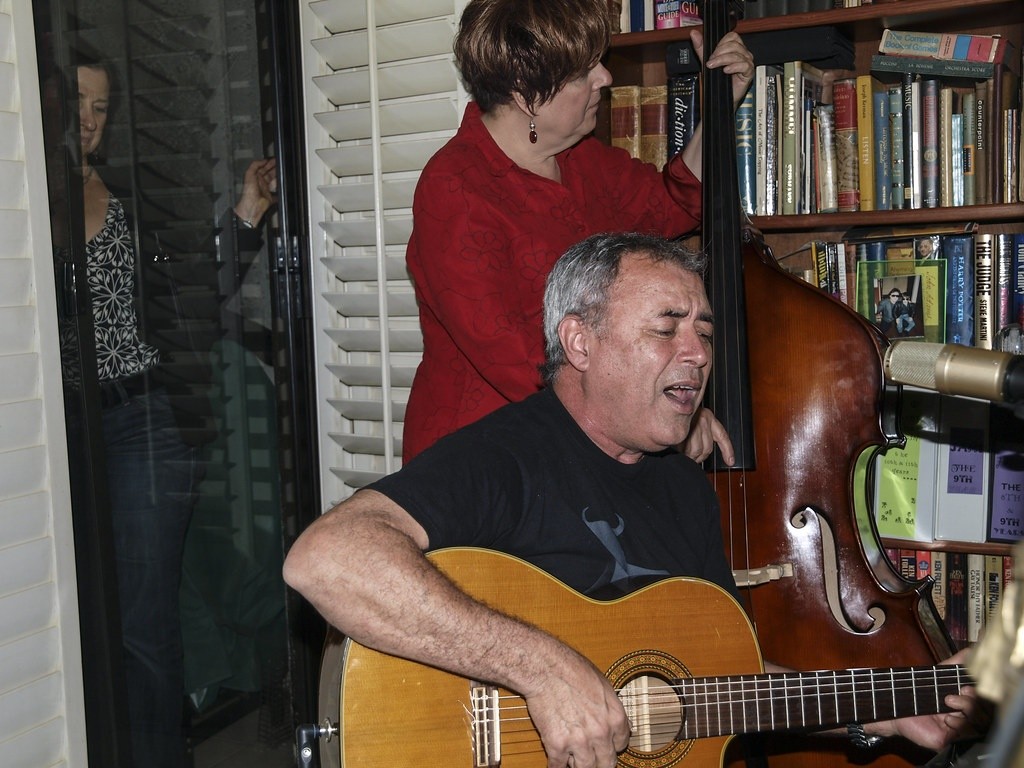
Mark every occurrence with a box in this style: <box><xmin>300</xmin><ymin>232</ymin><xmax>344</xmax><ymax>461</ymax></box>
<box><xmin>72</xmin><ymin>44</ymin><xmax>279</xmax><ymax>759</ymax></box>
<box><xmin>398</xmin><ymin>1</ymin><xmax>763</xmax><ymax>477</ymax></box>
<box><xmin>280</xmin><ymin>227</ymin><xmax>985</xmax><ymax>768</ymax></box>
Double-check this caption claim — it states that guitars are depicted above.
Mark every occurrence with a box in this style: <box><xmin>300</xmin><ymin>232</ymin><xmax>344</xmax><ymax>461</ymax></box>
<box><xmin>314</xmin><ymin>542</ymin><xmax>974</xmax><ymax>767</ymax></box>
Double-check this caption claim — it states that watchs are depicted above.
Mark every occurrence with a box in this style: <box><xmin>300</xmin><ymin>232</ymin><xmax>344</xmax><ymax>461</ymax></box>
<box><xmin>848</xmin><ymin>722</ymin><xmax>884</xmax><ymax>752</ymax></box>
<box><xmin>233</xmin><ymin>207</ymin><xmax>254</xmax><ymax>230</ymax></box>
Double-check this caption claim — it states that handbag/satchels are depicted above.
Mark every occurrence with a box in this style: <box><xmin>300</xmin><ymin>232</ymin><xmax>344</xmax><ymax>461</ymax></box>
<box><xmin>148</xmin><ymin>329</ymin><xmax>217</xmax><ymax>445</ymax></box>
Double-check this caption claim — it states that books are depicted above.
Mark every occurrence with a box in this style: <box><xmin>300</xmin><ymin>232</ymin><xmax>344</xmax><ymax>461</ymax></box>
<box><xmin>600</xmin><ymin>0</ymin><xmax>1024</xmax><ymax>647</ymax></box>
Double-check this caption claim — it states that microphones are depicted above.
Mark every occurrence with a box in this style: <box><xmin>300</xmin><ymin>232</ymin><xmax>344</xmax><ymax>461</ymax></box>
<box><xmin>883</xmin><ymin>339</ymin><xmax>1024</xmax><ymax>405</ymax></box>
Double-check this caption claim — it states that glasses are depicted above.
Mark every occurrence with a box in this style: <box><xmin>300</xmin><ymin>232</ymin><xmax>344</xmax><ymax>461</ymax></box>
<box><xmin>892</xmin><ymin>295</ymin><xmax>899</xmax><ymax>297</ymax></box>
<box><xmin>902</xmin><ymin>298</ymin><xmax>908</xmax><ymax>300</ymax></box>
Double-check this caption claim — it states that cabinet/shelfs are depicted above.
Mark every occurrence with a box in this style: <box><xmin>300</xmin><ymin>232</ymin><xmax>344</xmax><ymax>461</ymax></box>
<box><xmin>593</xmin><ymin>0</ymin><xmax>1024</xmax><ymax>654</ymax></box>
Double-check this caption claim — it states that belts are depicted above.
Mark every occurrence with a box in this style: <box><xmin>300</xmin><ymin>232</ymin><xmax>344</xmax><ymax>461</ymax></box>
<box><xmin>66</xmin><ymin>367</ymin><xmax>164</xmax><ymax>412</ymax></box>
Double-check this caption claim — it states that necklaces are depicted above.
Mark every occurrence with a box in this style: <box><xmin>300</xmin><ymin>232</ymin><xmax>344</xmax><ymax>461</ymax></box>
<box><xmin>81</xmin><ymin>165</ymin><xmax>95</xmax><ymax>185</ymax></box>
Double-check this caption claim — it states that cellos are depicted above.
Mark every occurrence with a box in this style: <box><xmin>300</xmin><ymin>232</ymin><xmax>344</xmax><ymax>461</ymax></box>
<box><xmin>684</xmin><ymin>1</ymin><xmax>956</xmax><ymax>768</ymax></box>
